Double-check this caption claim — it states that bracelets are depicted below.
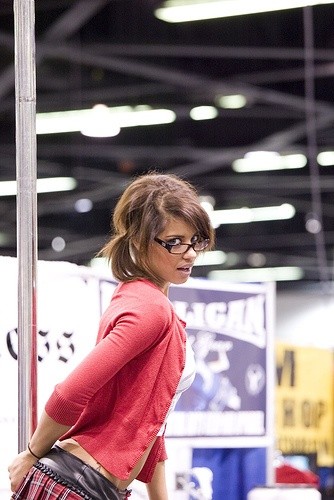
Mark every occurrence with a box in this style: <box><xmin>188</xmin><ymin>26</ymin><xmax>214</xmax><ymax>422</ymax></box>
<box><xmin>27</xmin><ymin>443</ymin><xmax>42</xmax><ymax>460</ymax></box>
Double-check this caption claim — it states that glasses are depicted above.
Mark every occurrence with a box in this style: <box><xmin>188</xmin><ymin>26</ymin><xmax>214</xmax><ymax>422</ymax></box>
<box><xmin>154</xmin><ymin>235</ymin><xmax>211</xmax><ymax>257</ymax></box>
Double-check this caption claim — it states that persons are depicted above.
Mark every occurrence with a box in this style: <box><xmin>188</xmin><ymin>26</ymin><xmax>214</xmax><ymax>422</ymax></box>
<box><xmin>8</xmin><ymin>174</ymin><xmax>219</xmax><ymax>500</ymax></box>
